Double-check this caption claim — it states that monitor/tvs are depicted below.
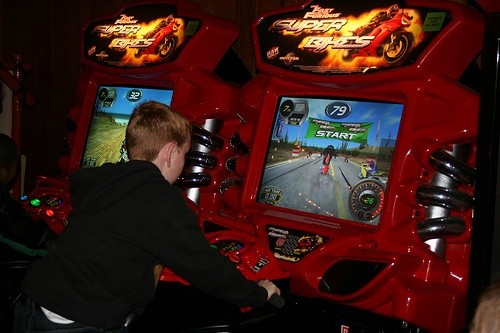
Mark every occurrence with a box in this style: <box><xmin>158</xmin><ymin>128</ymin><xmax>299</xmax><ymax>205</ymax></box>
<box><xmin>79</xmin><ymin>84</ymin><xmax>172</xmax><ymax>170</ymax></box>
<box><xmin>256</xmin><ymin>93</ymin><xmax>404</xmax><ymax>228</ymax></box>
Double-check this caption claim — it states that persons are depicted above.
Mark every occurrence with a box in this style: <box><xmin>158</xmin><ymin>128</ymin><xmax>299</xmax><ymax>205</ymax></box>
<box><xmin>26</xmin><ymin>100</ymin><xmax>282</xmax><ymax>333</ymax></box>
<box><xmin>0</xmin><ymin>132</ymin><xmax>52</xmax><ymax>333</ymax></box>
